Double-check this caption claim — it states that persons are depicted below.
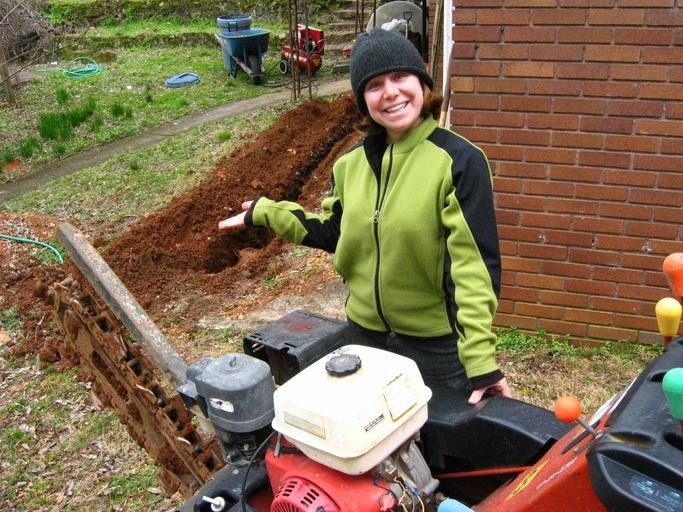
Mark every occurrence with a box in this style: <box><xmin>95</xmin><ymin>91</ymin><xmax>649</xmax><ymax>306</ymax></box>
<box><xmin>218</xmin><ymin>28</ymin><xmax>511</xmax><ymax>404</ymax></box>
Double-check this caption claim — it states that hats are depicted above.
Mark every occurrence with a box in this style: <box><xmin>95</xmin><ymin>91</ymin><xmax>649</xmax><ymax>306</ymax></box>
<box><xmin>349</xmin><ymin>29</ymin><xmax>435</xmax><ymax>118</ymax></box>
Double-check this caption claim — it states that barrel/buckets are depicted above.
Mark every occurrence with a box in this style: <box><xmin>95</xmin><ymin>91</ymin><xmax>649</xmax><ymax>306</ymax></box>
<box><xmin>216</xmin><ymin>13</ymin><xmax>253</xmax><ymax>69</ymax></box>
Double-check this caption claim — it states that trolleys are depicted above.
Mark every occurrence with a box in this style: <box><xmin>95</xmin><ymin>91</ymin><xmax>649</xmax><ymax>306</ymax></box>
<box><xmin>213</xmin><ymin>25</ymin><xmax>271</xmax><ymax>85</ymax></box>
